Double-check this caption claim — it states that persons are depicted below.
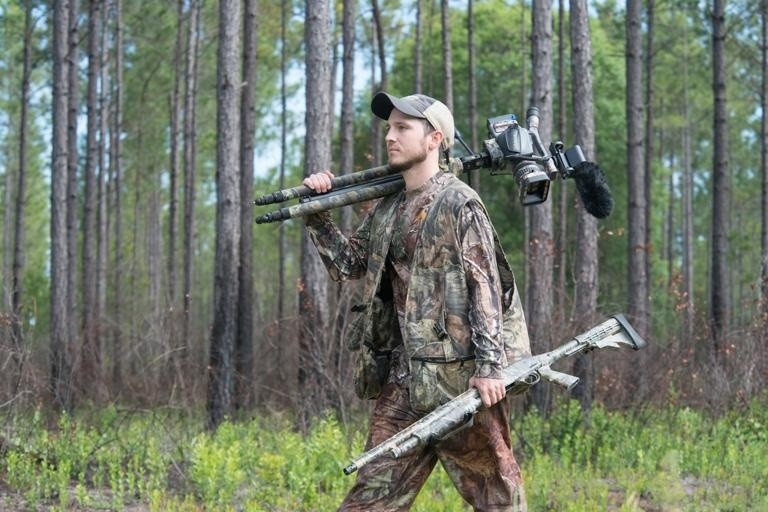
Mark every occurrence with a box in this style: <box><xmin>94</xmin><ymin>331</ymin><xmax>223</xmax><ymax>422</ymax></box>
<box><xmin>297</xmin><ymin>93</ymin><xmax>533</xmax><ymax>512</ymax></box>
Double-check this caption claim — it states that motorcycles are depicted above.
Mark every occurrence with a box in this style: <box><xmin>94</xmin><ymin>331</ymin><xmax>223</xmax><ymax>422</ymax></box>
<box><xmin>253</xmin><ymin>157</ymin><xmax>466</xmax><ymax>223</ymax></box>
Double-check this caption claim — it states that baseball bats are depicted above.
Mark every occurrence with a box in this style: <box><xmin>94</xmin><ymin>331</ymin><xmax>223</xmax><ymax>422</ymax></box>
<box><xmin>343</xmin><ymin>312</ymin><xmax>647</xmax><ymax>476</ymax></box>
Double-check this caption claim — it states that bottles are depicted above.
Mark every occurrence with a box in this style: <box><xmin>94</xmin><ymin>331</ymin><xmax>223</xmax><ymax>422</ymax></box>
<box><xmin>371</xmin><ymin>91</ymin><xmax>456</xmax><ymax>152</ymax></box>
